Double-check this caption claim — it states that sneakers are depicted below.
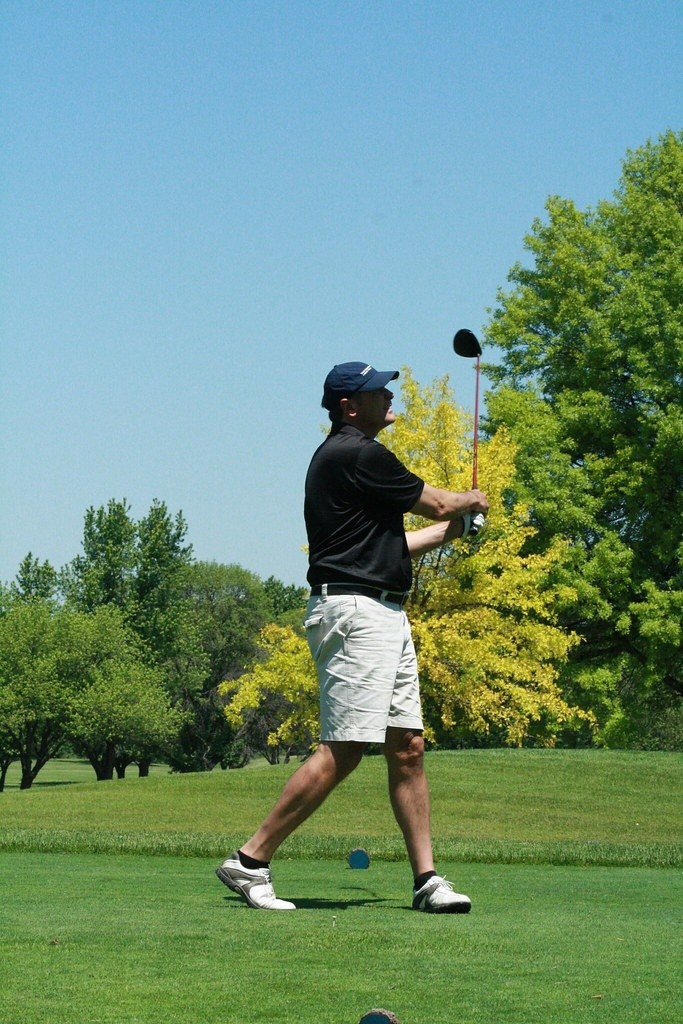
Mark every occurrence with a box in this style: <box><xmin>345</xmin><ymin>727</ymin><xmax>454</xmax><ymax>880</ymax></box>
<box><xmin>215</xmin><ymin>850</ymin><xmax>297</xmax><ymax>910</ymax></box>
<box><xmin>411</xmin><ymin>876</ymin><xmax>472</xmax><ymax>913</ymax></box>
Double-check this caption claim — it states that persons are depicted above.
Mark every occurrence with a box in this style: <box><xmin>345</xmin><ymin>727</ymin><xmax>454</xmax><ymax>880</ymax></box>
<box><xmin>213</xmin><ymin>361</ymin><xmax>490</xmax><ymax>913</ymax></box>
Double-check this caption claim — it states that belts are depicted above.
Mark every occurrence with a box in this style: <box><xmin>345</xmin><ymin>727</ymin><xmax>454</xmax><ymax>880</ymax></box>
<box><xmin>310</xmin><ymin>585</ymin><xmax>409</xmax><ymax>607</ymax></box>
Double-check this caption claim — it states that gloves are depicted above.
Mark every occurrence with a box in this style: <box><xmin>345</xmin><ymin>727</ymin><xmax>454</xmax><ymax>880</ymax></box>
<box><xmin>461</xmin><ymin>512</ymin><xmax>485</xmax><ymax>539</ymax></box>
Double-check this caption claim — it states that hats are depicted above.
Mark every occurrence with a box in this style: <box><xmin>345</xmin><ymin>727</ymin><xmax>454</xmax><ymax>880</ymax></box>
<box><xmin>323</xmin><ymin>362</ymin><xmax>400</xmax><ymax>412</ymax></box>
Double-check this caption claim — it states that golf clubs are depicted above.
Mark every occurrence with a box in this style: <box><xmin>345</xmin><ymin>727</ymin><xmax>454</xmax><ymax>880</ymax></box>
<box><xmin>451</xmin><ymin>327</ymin><xmax>483</xmax><ymax>535</ymax></box>
